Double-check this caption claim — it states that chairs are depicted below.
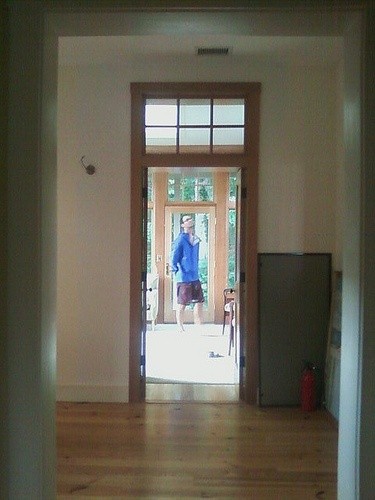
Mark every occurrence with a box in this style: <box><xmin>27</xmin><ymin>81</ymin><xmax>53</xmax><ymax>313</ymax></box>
<box><xmin>221</xmin><ymin>287</ymin><xmax>235</xmax><ymax>355</ymax></box>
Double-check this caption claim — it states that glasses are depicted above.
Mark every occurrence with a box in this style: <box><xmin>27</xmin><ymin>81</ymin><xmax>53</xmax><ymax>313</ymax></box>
<box><xmin>182</xmin><ymin>218</ymin><xmax>193</xmax><ymax>224</ymax></box>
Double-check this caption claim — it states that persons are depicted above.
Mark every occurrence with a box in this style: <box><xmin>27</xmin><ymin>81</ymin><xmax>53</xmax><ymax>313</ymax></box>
<box><xmin>170</xmin><ymin>214</ymin><xmax>205</xmax><ymax>334</ymax></box>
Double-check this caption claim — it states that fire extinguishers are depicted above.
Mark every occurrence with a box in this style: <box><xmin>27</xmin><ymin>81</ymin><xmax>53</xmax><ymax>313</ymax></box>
<box><xmin>300</xmin><ymin>357</ymin><xmax>320</xmax><ymax>411</ymax></box>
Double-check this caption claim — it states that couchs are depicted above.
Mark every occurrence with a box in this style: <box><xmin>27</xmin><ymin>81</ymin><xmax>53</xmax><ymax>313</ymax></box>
<box><xmin>147</xmin><ymin>273</ymin><xmax>159</xmax><ymax>332</ymax></box>
